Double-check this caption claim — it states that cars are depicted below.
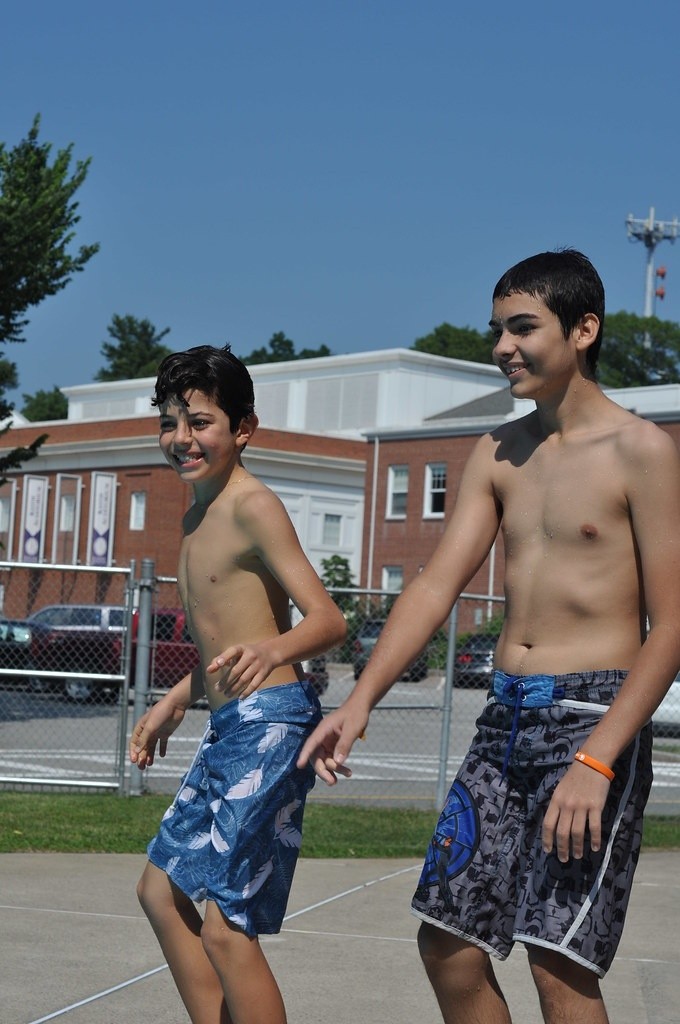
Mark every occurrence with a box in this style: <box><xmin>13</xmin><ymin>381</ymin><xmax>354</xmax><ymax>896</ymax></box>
<box><xmin>0</xmin><ymin>621</ymin><xmax>121</xmax><ymax>703</ymax></box>
<box><xmin>127</xmin><ymin>608</ymin><xmax>330</xmax><ymax>705</ymax></box>
<box><xmin>352</xmin><ymin>620</ymin><xmax>428</xmax><ymax>683</ymax></box>
<box><xmin>453</xmin><ymin>634</ymin><xmax>502</xmax><ymax>687</ymax></box>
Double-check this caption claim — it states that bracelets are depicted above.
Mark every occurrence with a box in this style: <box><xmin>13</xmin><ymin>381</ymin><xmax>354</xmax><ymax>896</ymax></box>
<box><xmin>574</xmin><ymin>752</ymin><xmax>614</xmax><ymax>779</ymax></box>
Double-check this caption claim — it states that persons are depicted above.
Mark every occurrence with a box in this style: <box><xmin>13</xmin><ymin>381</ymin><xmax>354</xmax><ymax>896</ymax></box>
<box><xmin>303</xmin><ymin>250</ymin><xmax>679</xmax><ymax>1024</ymax></box>
<box><xmin>126</xmin><ymin>346</ymin><xmax>346</xmax><ymax>1024</ymax></box>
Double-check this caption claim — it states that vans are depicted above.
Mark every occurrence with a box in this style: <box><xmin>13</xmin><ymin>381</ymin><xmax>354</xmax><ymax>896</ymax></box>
<box><xmin>24</xmin><ymin>605</ymin><xmax>127</xmax><ymax>635</ymax></box>
